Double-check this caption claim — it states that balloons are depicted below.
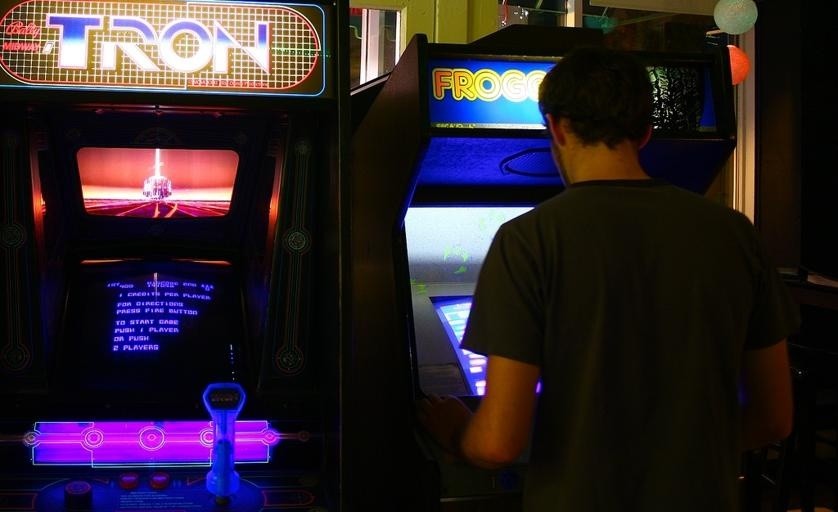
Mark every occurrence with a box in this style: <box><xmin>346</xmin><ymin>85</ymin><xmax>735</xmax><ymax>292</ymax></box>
<box><xmin>714</xmin><ymin>0</ymin><xmax>758</xmax><ymax>36</ymax></box>
<box><xmin>722</xmin><ymin>43</ymin><xmax>753</xmax><ymax>87</ymax></box>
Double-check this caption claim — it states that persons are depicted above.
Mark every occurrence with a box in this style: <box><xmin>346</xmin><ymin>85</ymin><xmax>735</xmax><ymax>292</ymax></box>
<box><xmin>412</xmin><ymin>43</ymin><xmax>796</xmax><ymax>510</ymax></box>
